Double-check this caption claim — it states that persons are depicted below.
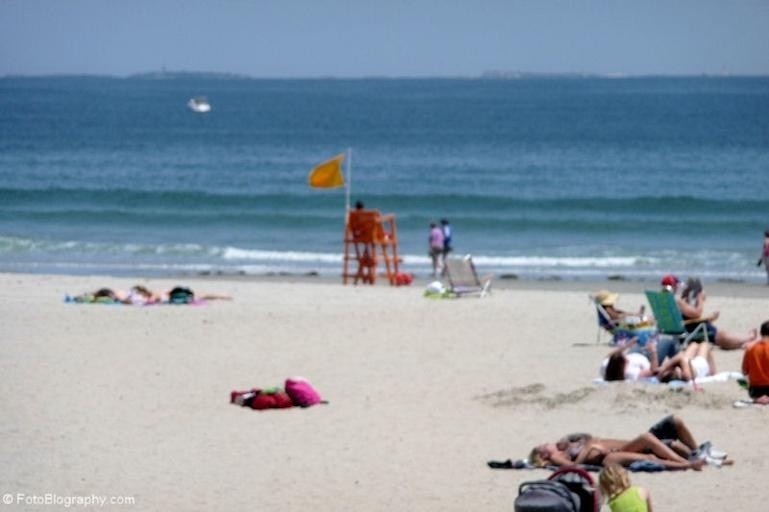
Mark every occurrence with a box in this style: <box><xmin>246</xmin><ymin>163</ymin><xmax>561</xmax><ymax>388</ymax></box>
<box><xmin>126</xmin><ymin>286</ymin><xmax>230</xmax><ymax>306</ymax></box>
<box><xmin>441</xmin><ymin>218</ymin><xmax>453</xmax><ymax>277</ymax></box>
<box><xmin>347</xmin><ymin>201</ymin><xmax>392</xmax><ymax>242</ymax></box>
<box><xmin>758</xmin><ymin>230</ymin><xmax>769</xmax><ymax>287</ymax></box>
<box><xmin>93</xmin><ymin>288</ymin><xmax>128</xmax><ymax>304</ymax></box>
<box><xmin>426</xmin><ymin>221</ymin><xmax>444</xmax><ymax>277</ymax></box>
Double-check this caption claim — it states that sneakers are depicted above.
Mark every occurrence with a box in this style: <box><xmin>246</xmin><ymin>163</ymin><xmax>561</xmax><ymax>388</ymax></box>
<box><xmin>690</xmin><ymin>440</ymin><xmax>734</xmax><ymax>468</ymax></box>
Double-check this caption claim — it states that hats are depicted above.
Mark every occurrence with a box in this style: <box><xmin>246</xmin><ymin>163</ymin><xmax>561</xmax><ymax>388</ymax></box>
<box><xmin>661</xmin><ymin>276</ymin><xmax>679</xmax><ymax>285</ymax></box>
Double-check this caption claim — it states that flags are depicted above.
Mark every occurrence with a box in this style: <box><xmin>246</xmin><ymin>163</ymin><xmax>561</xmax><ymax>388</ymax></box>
<box><xmin>310</xmin><ymin>152</ymin><xmax>345</xmax><ymax>190</ymax></box>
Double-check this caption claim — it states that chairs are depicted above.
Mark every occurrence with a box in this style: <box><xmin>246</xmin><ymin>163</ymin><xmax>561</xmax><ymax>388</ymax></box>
<box><xmin>343</xmin><ymin>208</ymin><xmax>401</xmax><ymax>285</ymax></box>
<box><xmin>588</xmin><ymin>284</ymin><xmax>713</xmax><ymax>366</ymax></box>
<box><xmin>440</xmin><ymin>255</ymin><xmax>496</xmax><ymax>298</ymax></box>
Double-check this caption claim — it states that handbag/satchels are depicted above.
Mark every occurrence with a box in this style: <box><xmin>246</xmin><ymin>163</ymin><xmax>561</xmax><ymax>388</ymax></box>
<box><xmin>231</xmin><ymin>379</ymin><xmax>292</xmax><ymax>410</ymax></box>
<box><xmin>285</xmin><ymin>376</ymin><xmax>321</xmax><ymax>408</ymax></box>
